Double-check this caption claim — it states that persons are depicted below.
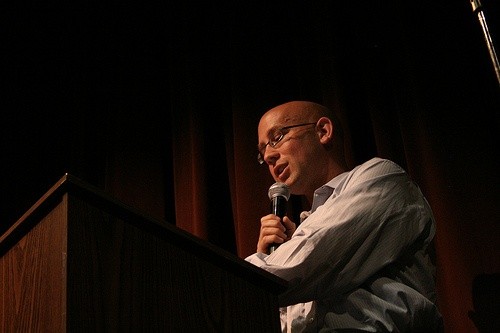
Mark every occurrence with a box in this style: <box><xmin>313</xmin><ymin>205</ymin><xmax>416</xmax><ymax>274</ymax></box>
<box><xmin>244</xmin><ymin>101</ymin><xmax>443</xmax><ymax>333</ymax></box>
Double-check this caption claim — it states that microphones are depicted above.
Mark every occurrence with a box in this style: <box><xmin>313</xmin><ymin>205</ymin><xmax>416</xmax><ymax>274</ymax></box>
<box><xmin>268</xmin><ymin>182</ymin><xmax>290</xmax><ymax>255</ymax></box>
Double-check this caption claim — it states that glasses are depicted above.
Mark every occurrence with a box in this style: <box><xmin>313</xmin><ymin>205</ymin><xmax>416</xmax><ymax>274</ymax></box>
<box><xmin>257</xmin><ymin>122</ymin><xmax>317</xmax><ymax>164</ymax></box>
